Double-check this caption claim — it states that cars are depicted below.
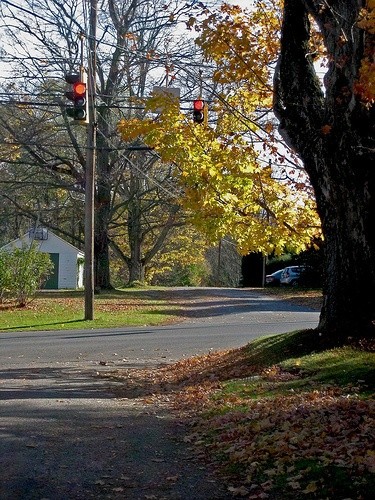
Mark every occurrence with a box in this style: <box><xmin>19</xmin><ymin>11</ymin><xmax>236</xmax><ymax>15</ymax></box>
<box><xmin>266</xmin><ymin>269</ymin><xmax>284</xmax><ymax>284</ymax></box>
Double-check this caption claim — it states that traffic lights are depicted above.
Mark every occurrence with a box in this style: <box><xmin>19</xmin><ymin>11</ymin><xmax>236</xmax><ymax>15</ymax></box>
<box><xmin>193</xmin><ymin>100</ymin><xmax>204</xmax><ymax>124</ymax></box>
<box><xmin>73</xmin><ymin>83</ymin><xmax>86</xmax><ymax>120</ymax></box>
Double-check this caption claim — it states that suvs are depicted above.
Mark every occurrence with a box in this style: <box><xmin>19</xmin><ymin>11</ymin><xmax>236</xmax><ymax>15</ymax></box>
<box><xmin>280</xmin><ymin>266</ymin><xmax>313</xmax><ymax>287</ymax></box>
